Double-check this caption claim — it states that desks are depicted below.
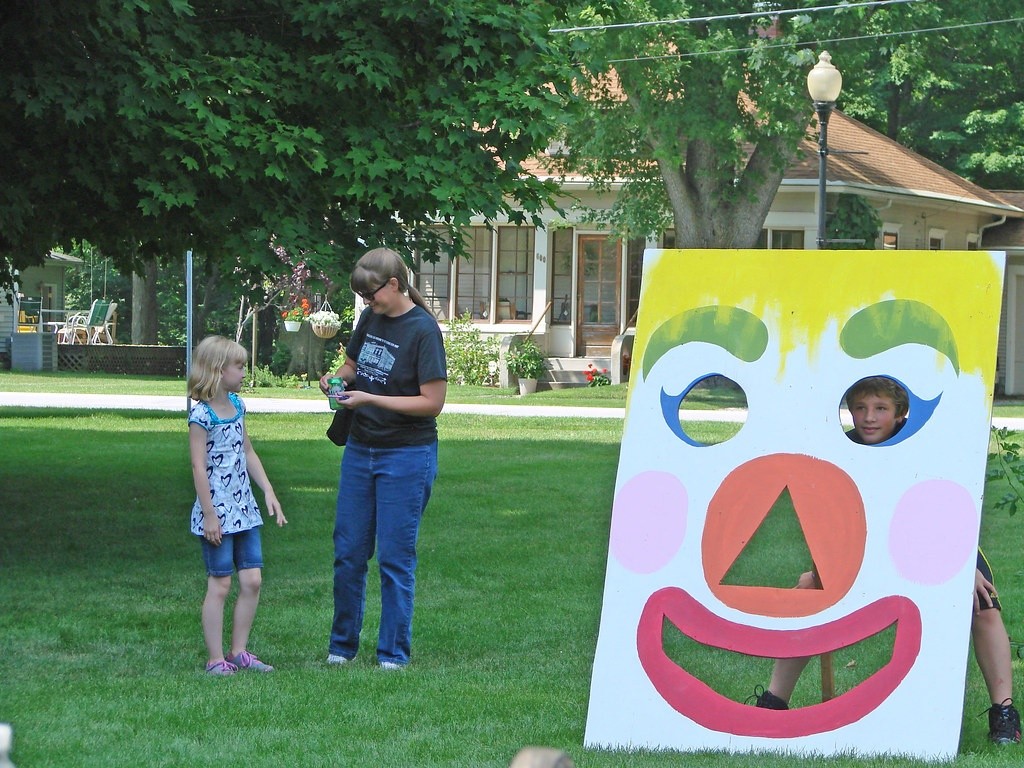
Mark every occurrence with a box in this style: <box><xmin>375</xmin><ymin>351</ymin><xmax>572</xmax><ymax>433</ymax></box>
<box><xmin>48</xmin><ymin>321</ymin><xmax>73</xmax><ymax>333</ymax></box>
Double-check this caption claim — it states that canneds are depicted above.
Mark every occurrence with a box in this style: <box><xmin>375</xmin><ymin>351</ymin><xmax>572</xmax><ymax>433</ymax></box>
<box><xmin>326</xmin><ymin>376</ymin><xmax>345</xmax><ymax>410</ymax></box>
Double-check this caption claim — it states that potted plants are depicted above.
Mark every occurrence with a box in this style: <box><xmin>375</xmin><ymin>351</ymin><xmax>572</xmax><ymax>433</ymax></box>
<box><xmin>502</xmin><ymin>337</ymin><xmax>548</xmax><ymax>395</ymax></box>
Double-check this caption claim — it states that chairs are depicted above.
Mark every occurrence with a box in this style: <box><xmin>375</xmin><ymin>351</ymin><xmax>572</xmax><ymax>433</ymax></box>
<box><xmin>58</xmin><ymin>297</ymin><xmax>117</xmax><ymax>346</ymax></box>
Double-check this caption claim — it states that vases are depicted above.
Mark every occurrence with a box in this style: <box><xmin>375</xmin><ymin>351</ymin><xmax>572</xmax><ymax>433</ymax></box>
<box><xmin>284</xmin><ymin>320</ymin><xmax>302</xmax><ymax>333</ymax></box>
<box><xmin>312</xmin><ymin>323</ymin><xmax>340</xmax><ymax>339</ymax></box>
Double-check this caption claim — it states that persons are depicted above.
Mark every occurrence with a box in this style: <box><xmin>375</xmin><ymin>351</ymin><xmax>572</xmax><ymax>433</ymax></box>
<box><xmin>318</xmin><ymin>248</ymin><xmax>447</xmax><ymax>670</ymax></box>
<box><xmin>744</xmin><ymin>376</ymin><xmax>1021</xmax><ymax>745</ymax></box>
<box><xmin>186</xmin><ymin>335</ymin><xmax>288</xmax><ymax>676</ymax></box>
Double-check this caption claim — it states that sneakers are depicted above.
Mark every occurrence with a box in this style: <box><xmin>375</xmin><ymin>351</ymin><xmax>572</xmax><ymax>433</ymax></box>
<box><xmin>978</xmin><ymin>698</ymin><xmax>1021</xmax><ymax>744</ymax></box>
<box><xmin>326</xmin><ymin>654</ymin><xmax>357</xmax><ymax>664</ymax></box>
<box><xmin>381</xmin><ymin>662</ymin><xmax>400</xmax><ymax>670</ymax></box>
<box><xmin>744</xmin><ymin>685</ymin><xmax>789</xmax><ymax>710</ymax></box>
<box><xmin>225</xmin><ymin>649</ymin><xmax>275</xmax><ymax>672</ymax></box>
<box><xmin>206</xmin><ymin>659</ymin><xmax>238</xmax><ymax>674</ymax></box>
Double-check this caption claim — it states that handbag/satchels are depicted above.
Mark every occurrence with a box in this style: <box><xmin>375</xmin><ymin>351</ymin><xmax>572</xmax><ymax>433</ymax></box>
<box><xmin>325</xmin><ymin>383</ymin><xmax>357</xmax><ymax>447</ymax></box>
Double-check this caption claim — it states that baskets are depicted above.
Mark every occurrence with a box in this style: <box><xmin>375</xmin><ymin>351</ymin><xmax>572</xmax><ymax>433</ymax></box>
<box><xmin>311</xmin><ymin>322</ymin><xmax>341</xmax><ymax>338</ymax></box>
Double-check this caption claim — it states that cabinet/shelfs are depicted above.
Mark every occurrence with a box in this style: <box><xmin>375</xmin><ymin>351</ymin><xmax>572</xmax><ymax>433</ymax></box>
<box><xmin>485</xmin><ymin>301</ymin><xmax>517</xmax><ymax>320</ymax></box>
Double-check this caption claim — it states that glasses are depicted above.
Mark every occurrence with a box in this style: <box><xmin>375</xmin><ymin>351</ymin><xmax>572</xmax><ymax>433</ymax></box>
<box><xmin>357</xmin><ymin>280</ymin><xmax>389</xmax><ymax>300</ymax></box>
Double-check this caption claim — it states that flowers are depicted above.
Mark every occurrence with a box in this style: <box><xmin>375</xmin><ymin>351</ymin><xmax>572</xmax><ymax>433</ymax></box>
<box><xmin>301</xmin><ymin>309</ymin><xmax>343</xmax><ymax>330</ymax></box>
<box><xmin>282</xmin><ymin>298</ymin><xmax>309</xmax><ymax>322</ymax></box>
